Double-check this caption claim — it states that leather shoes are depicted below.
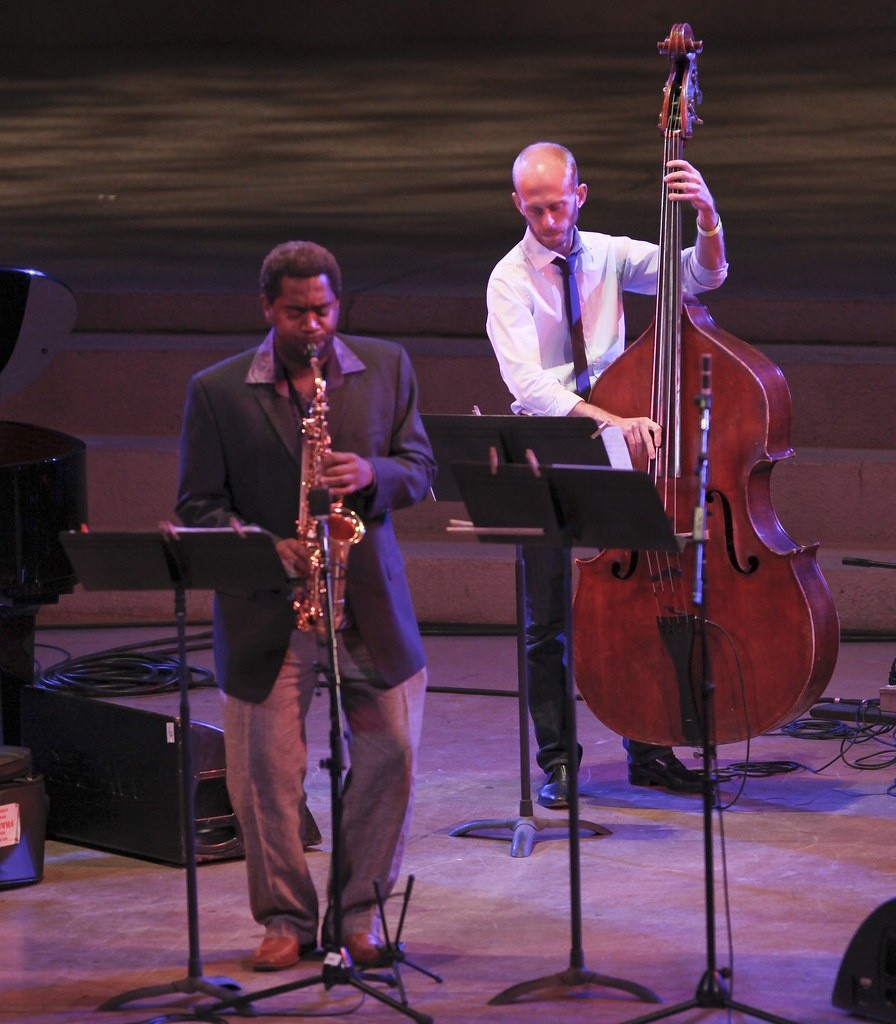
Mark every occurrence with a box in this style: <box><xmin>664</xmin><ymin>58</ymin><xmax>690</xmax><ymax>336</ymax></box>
<box><xmin>537</xmin><ymin>764</ymin><xmax>570</xmax><ymax>809</ymax></box>
<box><xmin>343</xmin><ymin>933</ymin><xmax>386</xmax><ymax>966</ymax></box>
<box><xmin>250</xmin><ymin>922</ymin><xmax>317</xmax><ymax>972</ymax></box>
<box><xmin>626</xmin><ymin>752</ymin><xmax>717</xmax><ymax>792</ymax></box>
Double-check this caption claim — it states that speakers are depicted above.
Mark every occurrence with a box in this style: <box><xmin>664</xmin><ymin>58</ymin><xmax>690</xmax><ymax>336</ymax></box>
<box><xmin>27</xmin><ymin>684</ymin><xmax>324</xmax><ymax>870</ymax></box>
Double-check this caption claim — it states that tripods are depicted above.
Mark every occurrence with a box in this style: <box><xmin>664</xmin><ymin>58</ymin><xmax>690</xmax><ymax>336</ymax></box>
<box><xmin>626</xmin><ymin>622</ymin><xmax>800</xmax><ymax>1024</ymax></box>
<box><xmin>449</xmin><ymin>457</ymin><xmax>682</xmax><ymax>859</ymax></box>
<box><xmin>65</xmin><ymin>527</ymin><xmax>293</xmax><ymax>1017</ymax></box>
<box><xmin>195</xmin><ymin>571</ymin><xmax>436</xmax><ymax>1024</ymax></box>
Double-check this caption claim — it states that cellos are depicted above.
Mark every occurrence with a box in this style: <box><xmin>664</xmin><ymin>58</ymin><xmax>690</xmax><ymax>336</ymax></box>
<box><xmin>567</xmin><ymin>22</ymin><xmax>842</xmax><ymax>749</ymax></box>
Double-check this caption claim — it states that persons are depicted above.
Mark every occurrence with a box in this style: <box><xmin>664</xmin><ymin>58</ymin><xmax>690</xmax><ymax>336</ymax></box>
<box><xmin>173</xmin><ymin>242</ymin><xmax>436</xmax><ymax>972</ymax></box>
<box><xmin>484</xmin><ymin>139</ymin><xmax>730</xmax><ymax>810</ymax></box>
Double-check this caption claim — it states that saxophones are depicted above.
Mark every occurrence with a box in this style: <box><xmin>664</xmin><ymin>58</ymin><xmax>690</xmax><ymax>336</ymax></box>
<box><xmin>290</xmin><ymin>349</ymin><xmax>366</xmax><ymax>635</ymax></box>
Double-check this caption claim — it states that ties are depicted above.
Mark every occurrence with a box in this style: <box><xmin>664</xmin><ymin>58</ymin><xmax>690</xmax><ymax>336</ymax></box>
<box><xmin>550</xmin><ymin>253</ymin><xmax>592</xmax><ymax>402</ymax></box>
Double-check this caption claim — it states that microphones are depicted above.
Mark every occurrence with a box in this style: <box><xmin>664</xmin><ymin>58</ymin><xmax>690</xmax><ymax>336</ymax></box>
<box><xmin>666</xmin><ymin>605</ymin><xmax>709</xmax><ymax>624</ymax></box>
<box><xmin>308</xmin><ymin>487</ymin><xmax>332</xmax><ymax>571</ymax></box>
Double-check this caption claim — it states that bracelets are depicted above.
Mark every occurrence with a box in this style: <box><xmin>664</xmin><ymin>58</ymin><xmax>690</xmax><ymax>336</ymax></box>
<box><xmin>695</xmin><ymin>213</ymin><xmax>723</xmax><ymax>238</ymax></box>
